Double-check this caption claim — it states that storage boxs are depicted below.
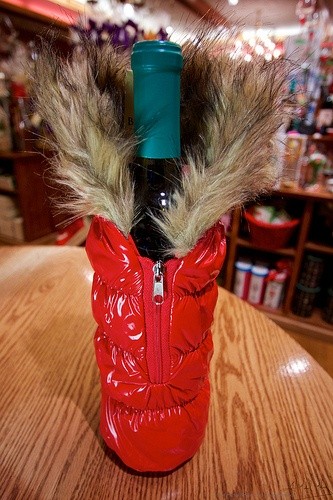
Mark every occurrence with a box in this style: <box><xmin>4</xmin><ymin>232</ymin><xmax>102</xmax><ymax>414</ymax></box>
<box><xmin>0</xmin><ymin>174</ymin><xmax>25</xmax><ymax>238</ymax></box>
<box><xmin>233</xmin><ymin>261</ymin><xmax>286</xmax><ymax>309</ymax></box>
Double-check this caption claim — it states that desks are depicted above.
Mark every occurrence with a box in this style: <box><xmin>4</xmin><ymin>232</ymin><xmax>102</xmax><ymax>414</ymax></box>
<box><xmin>0</xmin><ymin>244</ymin><xmax>333</xmax><ymax>500</ymax></box>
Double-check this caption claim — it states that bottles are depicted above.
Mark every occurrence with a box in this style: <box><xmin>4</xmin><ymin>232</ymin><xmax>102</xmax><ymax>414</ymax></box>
<box><xmin>123</xmin><ymin>38</ymin><xmax>189</xmax><ymax>263</ymax></box>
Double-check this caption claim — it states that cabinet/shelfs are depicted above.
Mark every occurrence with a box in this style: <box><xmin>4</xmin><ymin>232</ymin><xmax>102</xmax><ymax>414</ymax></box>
<box><xmin>222</xmin><ymin>169</ymin><xmax>333</xmax><ymax>338</ymax></box>
<box><xmin>0</xmin><ymin>92</ymin><xmax>64</xmax><ymax>245</ymax></box>
